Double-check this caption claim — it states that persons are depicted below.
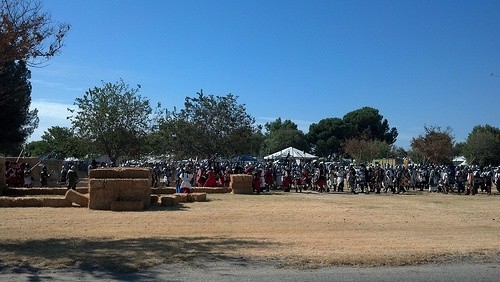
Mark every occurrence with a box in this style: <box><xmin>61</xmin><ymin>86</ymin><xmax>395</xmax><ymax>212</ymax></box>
<box><xmin>4</xmin><ymin>154</ymin><xmax>79</xmax><ymax>191</ymax></box>
<box><xmin>85</xmin><ymin>144</ymin><xmax>500</xmax><ymax>207</ymax></box>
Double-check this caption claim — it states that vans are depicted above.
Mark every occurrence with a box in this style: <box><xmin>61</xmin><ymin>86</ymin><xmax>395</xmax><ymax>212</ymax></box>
<box><xmin>366</xmin><ymin>157</ymin><xmax>404</xmax><ymax>168</ymax></box>
